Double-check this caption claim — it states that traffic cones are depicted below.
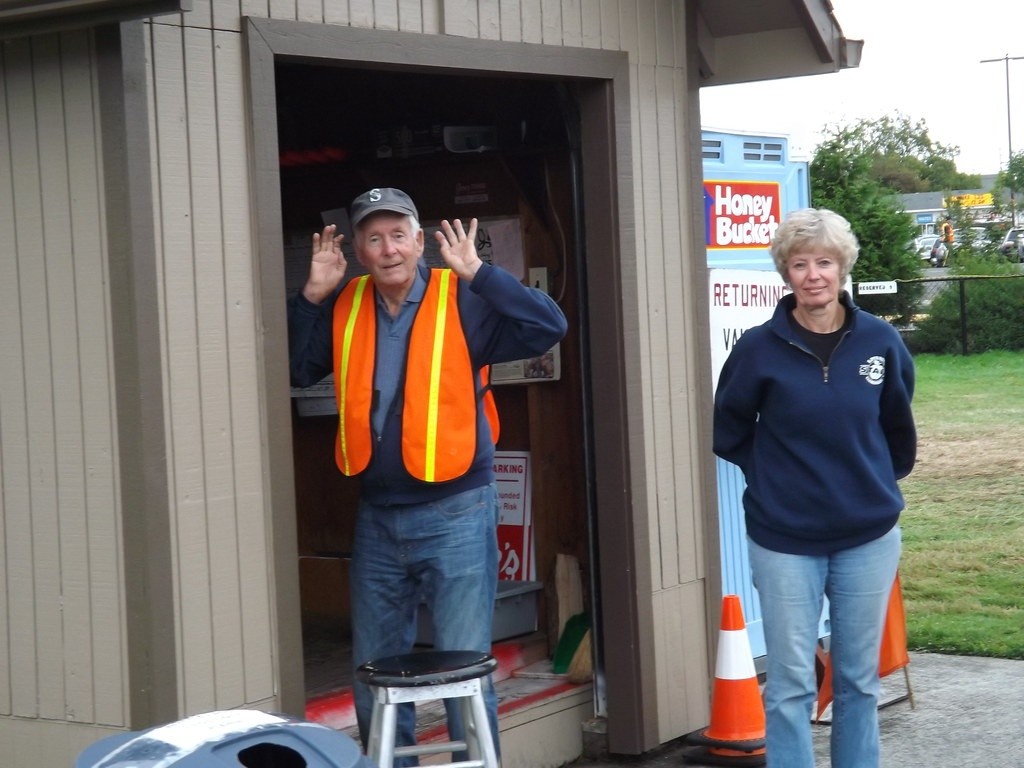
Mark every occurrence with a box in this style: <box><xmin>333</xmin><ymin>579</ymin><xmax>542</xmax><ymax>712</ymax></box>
<box><xmin>682</xmin><ymin>594</ymin><xmax>767</xmax><ymax>768</ymax></box>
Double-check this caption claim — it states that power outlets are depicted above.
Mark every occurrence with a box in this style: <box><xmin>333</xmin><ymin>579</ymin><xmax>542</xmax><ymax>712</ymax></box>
<box><xmin>528</xmin><ymin>267</ymin><xmax>548</xmax><ymax>294</ymax></box>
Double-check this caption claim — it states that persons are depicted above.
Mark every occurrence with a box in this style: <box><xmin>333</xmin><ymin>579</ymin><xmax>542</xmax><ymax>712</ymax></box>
<box><xmin>288</xmin><ymin>187</ymin><xmax>567</xmax><ymax>768</ymax></box>
<box><xmin>941</xmin><ymin>215</ymin><xmax>954</xmax><ymax>257</ymax></box>
<box><xmin>713</xmin><ymin>209</ymin><xmax>916</xmax><ymax>768</ymax></box>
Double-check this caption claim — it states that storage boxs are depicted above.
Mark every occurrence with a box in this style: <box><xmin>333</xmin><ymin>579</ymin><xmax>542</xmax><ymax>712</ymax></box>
<box><xmin>375</xmin><ymin>117</ymin><xmax>458</xmax><ymax>162</ymax></box>
<box><xmin>416</xmin><ymin>579</ymin><xmax>544</xmax><ymax>646</ymax></box>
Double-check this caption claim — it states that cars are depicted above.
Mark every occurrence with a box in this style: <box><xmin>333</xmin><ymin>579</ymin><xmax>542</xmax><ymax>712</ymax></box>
<box><xmin>910</xmin><ymin>214</ymin><xmax>1024</xmax><ymax>265</ymax></box>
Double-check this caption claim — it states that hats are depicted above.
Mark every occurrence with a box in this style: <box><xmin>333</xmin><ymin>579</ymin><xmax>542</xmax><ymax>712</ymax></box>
<box><xmin>351</xmin><ymin>188</ymin><xmax>419</xmax><ymax>226</ymax></box>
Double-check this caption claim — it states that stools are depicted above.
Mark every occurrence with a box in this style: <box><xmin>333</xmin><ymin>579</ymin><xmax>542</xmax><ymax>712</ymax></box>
<box><xmin>356</xmin><ymin>649</ymin><xmax>500</xmax><ymax>768</ymax></box>
